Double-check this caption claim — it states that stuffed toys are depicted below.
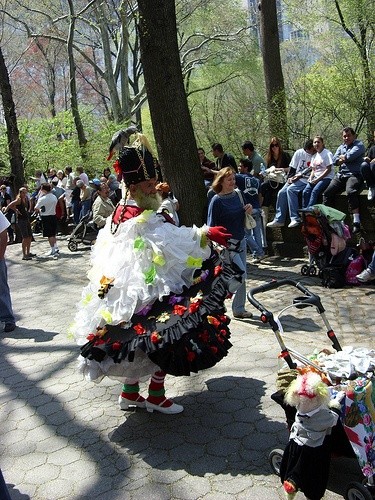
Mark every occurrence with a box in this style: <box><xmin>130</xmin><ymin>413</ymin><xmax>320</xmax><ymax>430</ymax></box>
<box><xmin>280</xmin><ymin>366</ymin><xmax>346</xmax><ymax>500</ymax></box>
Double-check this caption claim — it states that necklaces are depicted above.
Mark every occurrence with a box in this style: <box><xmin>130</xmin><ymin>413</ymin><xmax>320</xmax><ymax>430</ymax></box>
<box><xmin>360</xmin><ymin>130</ymin><xmax>375</xmax><ymax>200</ymax></box>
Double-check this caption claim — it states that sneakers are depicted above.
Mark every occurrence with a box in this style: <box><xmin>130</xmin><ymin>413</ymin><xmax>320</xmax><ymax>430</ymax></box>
<box><xmin>288</xmin><ymin>217</ymin><xmax>302</xmax><ymax>227</ymax></box>
<box><xmin>47</xmin><ymin>250</ymin><xmax>60</xmax><ymax>257</ymax></box>
<box><xmin>367</xmin><ymin>187</ymin><xmax>375</xmax><ymax>200</ymax></box>
<box><xmin>233</xmin><ymin>311</ymin><xmax>252</xmax><ymax>317</ymax></box>
<box><xmin>267</xmin><ymin>219</ymin><xmax>284</xmax><ymax>227</ymax></box>
<box><xmin>251</xmin><ymin>253</ymin><xmax>265</xmax><ymax>263</ymax></box>
<box><xmin>356</xmin><ymin>268</ymin><xmax>375</xmax><ymax>282</ymax></box>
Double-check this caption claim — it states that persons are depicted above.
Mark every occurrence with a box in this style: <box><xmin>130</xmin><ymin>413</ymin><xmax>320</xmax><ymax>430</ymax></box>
<box><xmin>302</xmin><ymin>136</ymin><xmax>335</xmax><ymax>232</ymax></box>
<box><xmin>241</xmin><ymin>140</ymin><xmax>266</xmax><ymax>178</ymax></box>
<box><xmin>260</xmin><ymin>137</ymin><xmax>291</xmax><ymax>211</ymax></box>
<box><xmin>92</xmin><ymin>183</ymin><xmax>115</xmax><ymax>235</ymax></box>
<box><xmin>266</xmin><ymin>139</ymin><xmax>317</xmax><ymax>227</ymax></box>
<box><xmin>211</xmin><ymin>143</ymin><xmax>239</xmax><ymax>173</ymax></box>
<box><xmin>155</xmin><ymin>183</ymin><xmax>180</xmax><ymax>226</ymax></box>
<box><xmin>207</xmin><ymin>167</ymin><xmax>253</xmax><ymax>318</ymax></box>
<box><xmin>29</xmin><ymin>166</ymin><xmax>122</xmax><ymax>236</ymax></box>
<box><xmin>104</xmin><ymin>146</ymin><xmax>231</xmax><ymax>414</ymax></box>
<box><xmin>197</xmin><ymin>147</ymin><xmax>216</xmax><ymax>190</ymax></box>
<box><xmin>0</xmin><ymin>211</ymin><xmax>16</xmax><ymax>332</ymax></box>
<box><xmin>201</xmin><ymin>159</ymin><xmax>265</xmax><ymax>263</ymax></box>
<box><xmin>323</xmin><ymin>127</ymin><xmax>366</xmax><ymax>233</ymax></box>
<box><xmin>8</xmin><ymin>187</ymin><xmax>36</xmax><ymax>260</ymax></box>
<box><xmin>355</xmin><ymin>252</ymin><xmax>375</xmax><ymax>283</ymax></box>
<box><xmin>34</xmin><ymin>183</ymin><xmax>60</xmax><ymax>260</ymax></box>
<box><xmin>0</xmin><ymin>185</ymin><xmax>14</xmax><ymax>245</ymax></box>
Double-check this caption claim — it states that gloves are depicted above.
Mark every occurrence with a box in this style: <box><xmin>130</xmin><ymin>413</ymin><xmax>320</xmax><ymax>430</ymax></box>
<box><xmin>207</xmin><ymin>226</ymin><xmax>232</xmax><ymax>248</ymax></box>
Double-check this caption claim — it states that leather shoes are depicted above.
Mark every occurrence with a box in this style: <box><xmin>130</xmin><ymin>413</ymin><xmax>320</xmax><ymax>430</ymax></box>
<box><xmin>5</xmin><ymin>323</ymin><xmax>14</xmax><ymax>332</ymax></box>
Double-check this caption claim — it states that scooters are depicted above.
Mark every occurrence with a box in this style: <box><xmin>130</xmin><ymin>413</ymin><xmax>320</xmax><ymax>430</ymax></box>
<box><xmin>67</xmin><ymin>211</ymin><xmax>97</xmax><ymax>251</ymax></box>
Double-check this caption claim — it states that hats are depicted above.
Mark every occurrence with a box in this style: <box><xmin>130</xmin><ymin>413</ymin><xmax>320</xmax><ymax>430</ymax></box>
<box><xmin>89</xmin><ymin>178</ymin><xmax>100</xmax><ymax>185</ymax></box>
<box><xmin>155</xmin><ymin>182</ymin><xmax>170</xmax><ymax>192</ymax></box>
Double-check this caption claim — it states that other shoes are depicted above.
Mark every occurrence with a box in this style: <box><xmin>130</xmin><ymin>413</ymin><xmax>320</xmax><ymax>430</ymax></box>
<box><xmin>29</xmin><ymin>253</ymin><xmax>36</xmax><ymax>257</ymax></box>
<box><xmin>352</xmin><ymin>222</ymin><xmax>360</xmax><ymax>233</ymax></box>
<box><xmin>22</xmin><ymin>256</ymin><xmax>32</xmax><ymax>260</ymax></box>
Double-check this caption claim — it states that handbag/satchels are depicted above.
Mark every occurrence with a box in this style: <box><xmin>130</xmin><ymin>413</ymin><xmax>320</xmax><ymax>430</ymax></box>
<box><xmin>234</xmin><ymin>189</ymin><xmax>256</xmax><ymax>229</ymax></box>
<box><xmin>266</xmin><ymin>171</ymin><xmax>286</xmax><ymax>188</ymax></box>
<box><xmin>319</xmin><ymin>265</ymin><xmax>345</xmax><ymax>288</ymax></box>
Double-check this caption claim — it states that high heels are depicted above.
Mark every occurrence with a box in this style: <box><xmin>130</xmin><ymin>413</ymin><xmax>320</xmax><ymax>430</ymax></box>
<box><xmin>145</xmin><ymin>398</ymin><xmax>184</xmax><ymax>414</ymax></box>
<box><xmin>119</xmin><ymin>395</ymin><xmax>146</xmax><ymax>409</ymax></box>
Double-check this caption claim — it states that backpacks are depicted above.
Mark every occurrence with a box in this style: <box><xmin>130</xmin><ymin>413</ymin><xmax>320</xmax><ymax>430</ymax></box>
<box><xmin>271</xmin><ymin>144</ymin><xmax>278</xmax><ymax>147</ymax></box>
<box><xmin>345</xmin><ymin>255</ymin><xmax>363</xmax><ymax>286</ymax></box>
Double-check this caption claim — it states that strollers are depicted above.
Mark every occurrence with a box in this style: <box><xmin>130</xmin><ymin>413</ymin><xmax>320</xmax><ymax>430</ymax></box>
<box><xmin>247</xmin><ymin>279</ymin><xmax>375</xmax><ymax>500</ymax></box>
<box><xmin>296</xmin><ymin>209</ymin><xmax>365</xmax><ymax>277</ymax></box>
